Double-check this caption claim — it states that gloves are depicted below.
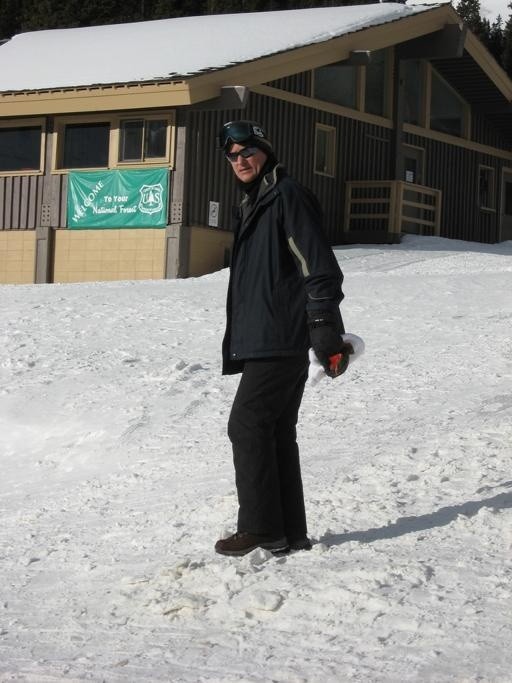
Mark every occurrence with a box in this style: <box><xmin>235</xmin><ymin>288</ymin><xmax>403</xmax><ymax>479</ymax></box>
<box><xmin>305</xmin><ymin>309</ymin><xmax>352</xmax><ymax>379</ymax></box>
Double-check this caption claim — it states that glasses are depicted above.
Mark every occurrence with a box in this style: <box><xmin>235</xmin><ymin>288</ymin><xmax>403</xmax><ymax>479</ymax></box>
<box><xmin>220</xmin><ymin>120</ymin><xmax>274</xmax><ymax>149</ymax></box>
<box><xmin>223</xmin><ymin>144</ymin><xmax>255</xmax><ymax>163</ymax></box>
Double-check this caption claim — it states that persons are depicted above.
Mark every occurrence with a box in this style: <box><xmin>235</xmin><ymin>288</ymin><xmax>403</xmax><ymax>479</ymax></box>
<box><xmin>215</xmin><ymin>119</ymin><xmax>349</xmax><ymax>558</ymax></box>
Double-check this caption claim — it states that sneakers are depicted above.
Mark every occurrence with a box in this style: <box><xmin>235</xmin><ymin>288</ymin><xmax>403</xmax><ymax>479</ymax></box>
<box><xmin>287</xmin><ymin>531</ymin><xmax>312</xmax><ymax>549</ymax></box>
<box><xmin>212</xmin><ymin>528</ymin><xmax>290</xmax><ymax>557</ymax></box>
